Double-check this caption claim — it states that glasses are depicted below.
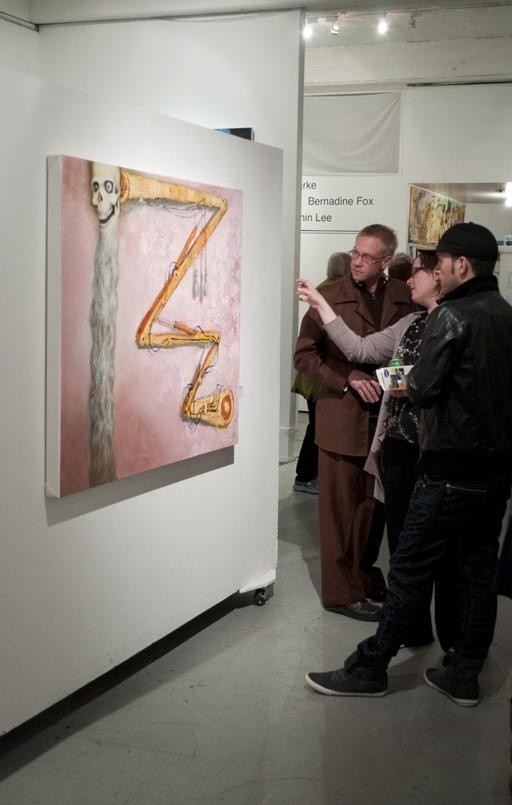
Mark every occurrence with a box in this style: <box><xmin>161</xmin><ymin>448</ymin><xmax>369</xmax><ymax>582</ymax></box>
<box><xmin>349</xmin><ymin>249</ymin><xmax>384</xmax><ymax>263</ymax></box>
<box><xmin>411</xmin><ymin>266</ymin><xmax>425</xmax><ymax>276</ymax></box>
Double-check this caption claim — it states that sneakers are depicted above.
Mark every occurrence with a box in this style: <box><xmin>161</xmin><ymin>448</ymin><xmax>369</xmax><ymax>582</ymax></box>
<box><xmin>304</xmin><ymin>665</ymin><xmax>388</xmax><ymax>699</ymax></box>
<box><xmin>292</xmin><ymin>479</ymin><xmax>320</xmax><ymax>495</ymax></box>
<box><xmin>422</xmin><ymin>648</ymin><xmax>487</xmax><ymax>707</ymax></box>
<box><xmin>367</xmin><ymin>591</ymin><xmax>388</xmax><ymax>603</ymax></box>
<box><xmin>327</xmin><ymin>601</ymin><xmax>382</xmax><ymax>622</ymax></box>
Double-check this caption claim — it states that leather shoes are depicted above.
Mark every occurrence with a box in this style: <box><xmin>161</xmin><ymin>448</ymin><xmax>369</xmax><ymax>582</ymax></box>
<box><xmin>355</xmin><ymin>629</ymin><xmax>435</xmax><ymax>653</ymax></box>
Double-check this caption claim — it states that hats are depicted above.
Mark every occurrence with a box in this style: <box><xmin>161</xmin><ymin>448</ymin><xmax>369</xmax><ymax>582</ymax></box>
<box><xmin>416</xmin><ymin>222</ymin><xmax>499</xmax><ymax>258</ymax></box>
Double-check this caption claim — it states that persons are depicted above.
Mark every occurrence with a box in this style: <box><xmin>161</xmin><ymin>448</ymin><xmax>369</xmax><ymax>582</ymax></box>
<box><xmin>289</xmin><ymin>220</ymin><xmax>512</xmax><ymax>650</ymax></box>
<box><xmin>301</xmin><ymin>223</ymin><xmax>512</xmax><ymax>709</ymax></box>
